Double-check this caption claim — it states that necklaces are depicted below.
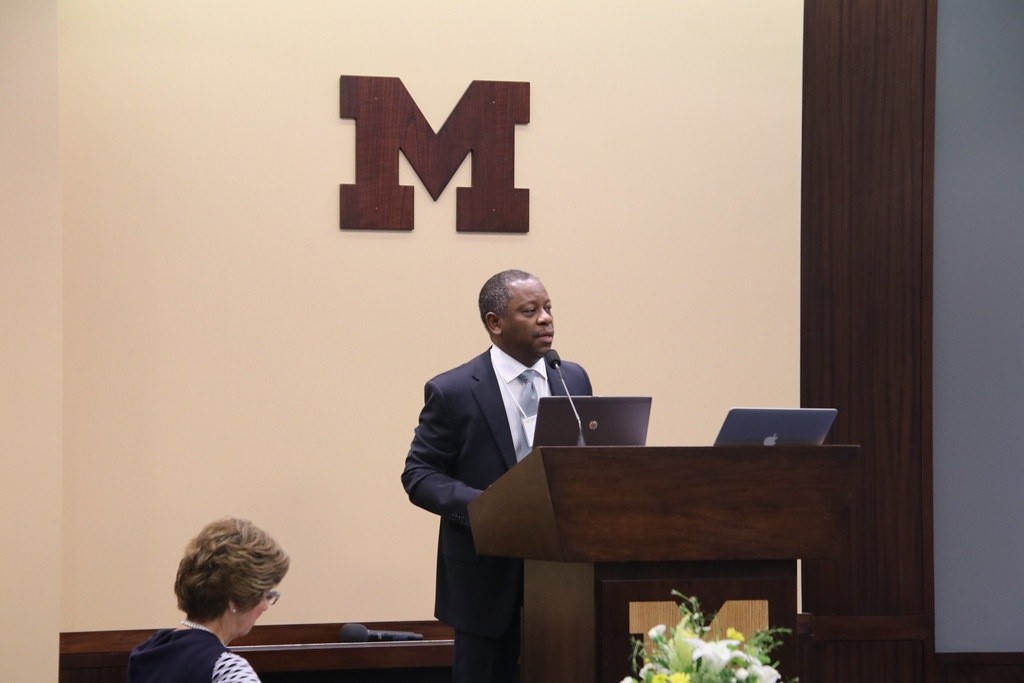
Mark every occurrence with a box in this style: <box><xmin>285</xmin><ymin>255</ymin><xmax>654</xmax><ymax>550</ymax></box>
<box><xmin>179</xmin><ymin>619</ymin><xmax>212</xmax><ymax>632</ymax></box>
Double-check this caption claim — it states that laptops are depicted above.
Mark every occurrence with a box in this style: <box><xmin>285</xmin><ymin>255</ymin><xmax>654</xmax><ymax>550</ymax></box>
<box><xmin>532</xmin><ymin>396</ymin><xmax>652</xmax><ymax>450</ymax></box>
<box><xmin>714</xmin><ymin>407</ymin><xmax>838</xmax><ymax>448</ymax></box>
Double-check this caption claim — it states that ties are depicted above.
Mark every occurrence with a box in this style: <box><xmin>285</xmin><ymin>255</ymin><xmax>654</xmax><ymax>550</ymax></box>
<box><xmin>517</xmin><ymin>369</ymin><xmax>538</xmax><ymax>416</ymax></box>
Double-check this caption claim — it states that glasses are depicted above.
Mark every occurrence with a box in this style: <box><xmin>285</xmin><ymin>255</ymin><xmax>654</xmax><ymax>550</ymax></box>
<box><xmin>263</xmin><ymin>591</ymin><xmax>280</xmax><ymax>604</ymax></box>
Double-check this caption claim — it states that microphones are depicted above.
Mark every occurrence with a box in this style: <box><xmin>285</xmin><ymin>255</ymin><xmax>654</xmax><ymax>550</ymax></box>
<box><xmin>546</xmin><ymin>349</ymin><xmax>585</xmax><ymax>447</ymax></box>
<box><xmin>340</xmin><ymin>624</ymin><xmax>423</xmax><ymax>643</ymax></box>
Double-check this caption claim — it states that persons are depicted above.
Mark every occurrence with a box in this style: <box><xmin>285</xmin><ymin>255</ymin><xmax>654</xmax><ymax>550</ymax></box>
<box><xmin>402</xmin><ymin>270</ymin><xmax>593</xmax><ymax>683</ymax></box>
<box><xmin>124</xmin><ymin>519</ymin><xmax>290</xmax><ymax>683</ymax></box>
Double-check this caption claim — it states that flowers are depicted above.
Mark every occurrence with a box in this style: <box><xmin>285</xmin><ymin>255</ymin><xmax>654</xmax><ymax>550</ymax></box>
<box><xmin>620</xmin><ymin>588</ymin><xmax>801</xmax><ymax>683</ymax></box>
